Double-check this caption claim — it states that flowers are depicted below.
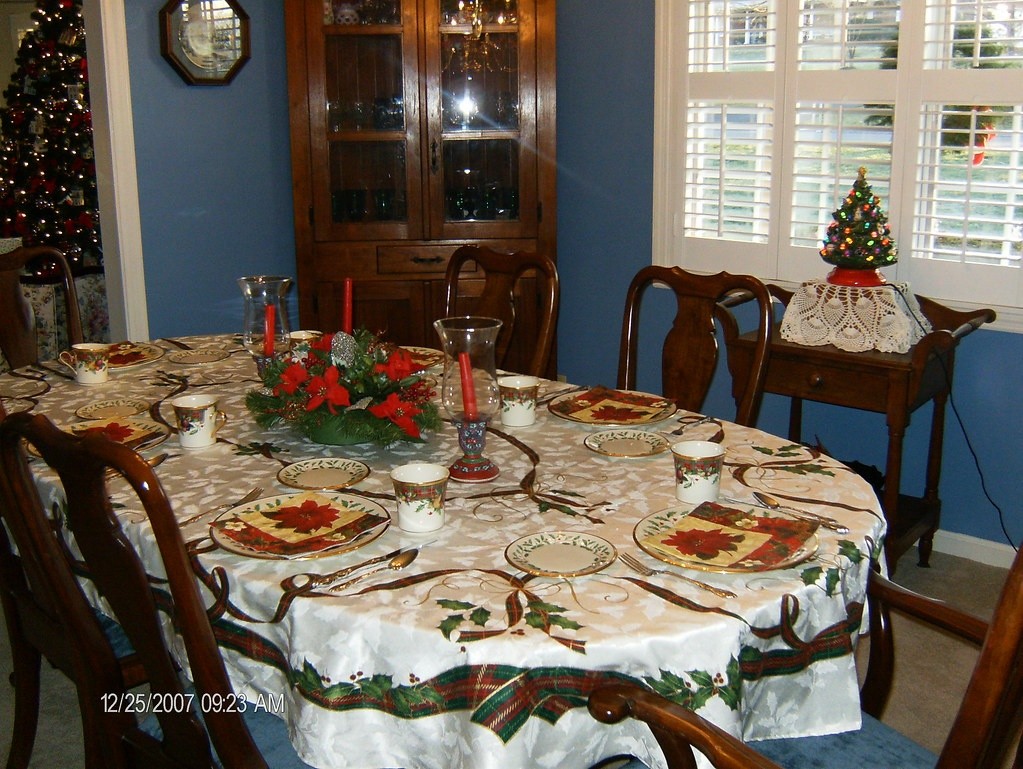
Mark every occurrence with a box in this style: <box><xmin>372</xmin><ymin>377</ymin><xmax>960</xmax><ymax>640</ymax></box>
<box><xmin>245</xmin><ymin>329</ymin><xmax>444</xmax><ymax>453</ymax></box>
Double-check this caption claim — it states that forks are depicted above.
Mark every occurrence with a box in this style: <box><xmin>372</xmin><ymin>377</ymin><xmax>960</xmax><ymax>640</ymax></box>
<box><xmin>178</xmin><ymin>487</ymin><xmax>264</xmax><ymax>528</ymax></box>
<box><xmin>660</xmin><ymin>416</ymin><xmax>713</xmax><ymax>438</ymax></box>
<box><xmin>618</xmin><ymin>552</ymin><xmax>739</xmax><ymax>599</ymax></box>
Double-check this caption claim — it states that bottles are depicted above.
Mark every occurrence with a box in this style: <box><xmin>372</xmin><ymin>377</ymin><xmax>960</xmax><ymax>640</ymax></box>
<box><xmin>322</xmin><ymin>0</ymin><xmax>400</xmax><ymax>26</ymax></box>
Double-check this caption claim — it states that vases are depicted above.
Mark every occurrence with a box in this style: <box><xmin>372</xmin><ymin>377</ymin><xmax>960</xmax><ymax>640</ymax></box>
<box><xmin>304</xmin><ymin>410</ymin><xmax>375</xmax><ymax>446</ymax></box>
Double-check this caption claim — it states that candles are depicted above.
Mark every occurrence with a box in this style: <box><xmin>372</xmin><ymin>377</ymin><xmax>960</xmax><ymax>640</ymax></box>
<box><xmin>343</xmin><ymin>277</ymin><xmax>353</xmax><ymax>336</ymax></box>
<box><xmin>236</xmin><ymin>276</ymin><xmax>292</xmax><ymax>387</ymax></box>
<box><xmin>434</xmin><ymin>316</ymin><xmax>504</xmax><ymax>483</ymax></box>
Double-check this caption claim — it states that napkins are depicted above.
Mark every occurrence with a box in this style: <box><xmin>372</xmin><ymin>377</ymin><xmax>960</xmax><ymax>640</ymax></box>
<box><xmin>97</xmin><ymin>340</ymin><xmax>152</xmax><ymax>368</ymax></box>
<box><xmin>208</xmin><ymin>486</ymin><xmax>392</xmax><ymax>560</ymax></box>
<box><xmin>548</xmin><ymin>385</ymin><xmax>681</xmax><ymax>425</ymax></box>
<box><xmin>642</xmin><ymin>501</ymin><xmax>821</xmax><ymax>572</ymax></box>
<box><xmin>65</xmin><ymin>415</ymin><xmax>166</xmax><ymax>451</ymax></box>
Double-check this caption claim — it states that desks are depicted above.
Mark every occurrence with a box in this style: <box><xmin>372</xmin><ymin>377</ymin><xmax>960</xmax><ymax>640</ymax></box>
<box><xmin>713</xmin><ymin>285</ymin><xmax>997</xmax><ymax>576</ymax></box>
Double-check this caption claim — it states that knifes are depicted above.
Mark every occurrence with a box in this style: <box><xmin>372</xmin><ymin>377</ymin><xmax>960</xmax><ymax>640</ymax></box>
<box><xmin>309</xmin><ymin>538</ymin><xmax>437</xmax><ymax>588</ymax></box>
<box><xmin>725</xmin><ymin>498</ymin><xmax>849</xmax><ymax>534</ymax></box>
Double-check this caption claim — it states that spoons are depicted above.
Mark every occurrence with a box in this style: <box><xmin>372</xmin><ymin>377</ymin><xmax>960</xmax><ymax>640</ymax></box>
<box><xmin>327</xmin><ymin>549</ymin><xmax>418</xmax><ymax>592</ymax></box>
<box><xmin>752</xmin><ymin>490</ymin><xmax>838</xmax><ymax>523</ymax></box>
<box><xmin>105</xmin><ymin>453</ymin><xmax>168</xmax><ymax>485</ymax></box>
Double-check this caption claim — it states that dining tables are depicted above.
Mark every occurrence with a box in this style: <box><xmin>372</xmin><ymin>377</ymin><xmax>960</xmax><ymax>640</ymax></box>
<box><xmin>0</xmin><ymin>332</ymin><xmax>895</xmax><ymax>769</ymax></box>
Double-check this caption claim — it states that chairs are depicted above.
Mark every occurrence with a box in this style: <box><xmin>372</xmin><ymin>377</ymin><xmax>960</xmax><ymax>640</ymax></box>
<box><xmin>0</xmin><ymin>404</ymin><xmax>183</xmax><ymax>769</ymax></box>
<box><xmin>617</xmin><ymin>264</ymin><xmax>775</xmax><ymax>428</ymax></box>
<box><xmin>0</xmin><ymin>409</ymin><xmax>315</xmax><ymax>769</ymax></box>
<box><xmin>442</xmin><ymin>243</ymin><xmax>559</xmax><ymax>376</ymax></box>
<box><xmin>586</xmin><ymin>540</ymin><xmax>1023</xmax><ymax>769</ymax></box>
<box><xmin>0</xmin><ymin>247</ymin><xmax>85</xmax><ymax>370</ymax></box>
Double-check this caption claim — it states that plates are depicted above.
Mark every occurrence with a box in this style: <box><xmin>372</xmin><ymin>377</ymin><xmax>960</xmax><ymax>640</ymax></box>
<box><xmin>632</xmin><ymin>502</ymin><xmax>819</xmax><ymax>574</ymax></box>
<box><xmin>584</xmin><ymin>429</ymin><xmax>670</xmax><ymax>457</ymax></box>
<box><xmin>504</xmin><ymin>531</ymin><xmax>617</xmax><ymax>577</ymax></box>
<box><xmin>548</xmin><ymin>389</ymin><xmax>677</xmax><ymax>427</ymax></box>
<box><xmin>208</xmin><ymin>493</ymin><xmax>392</xmax><ymax>561</ymax></box>
<box><xmin>27</xmin><ymin>418</ymin><xmax>171</xmax><ymax>458</ymax></box>
<box><xmin>276</xmin><ymin>457</ymin><xmax>371</xmax><ymax>491</ymax></box>
<box><xmin>75</xmin><ymin>398</ymin><xmax>151</xmax><ymax>420</ymax></box>
<box><xmin>400</xmin><ymin>345</ymin><xmax>454</xmax><ymax>381</ymax></box>
<box><xmin>56</xmin><ymin>342</ymin><xmax>166</xmax><ymax>372</ymax></box>
<box><xmin>167</xmin><ymin>348</ymin><xmax>231</xmax><ymax>366</ymax></box>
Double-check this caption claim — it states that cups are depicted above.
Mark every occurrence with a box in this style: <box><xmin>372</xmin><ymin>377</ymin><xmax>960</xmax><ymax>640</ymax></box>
<box><xmin>331</xmin><ymin>90</ymin><xmax>520</xmax><ymax>221</ymax></box>
<box><xmin>286</xmin><ymin>329</ymin><xmax>323</xmax><ymax>355</ymax></box>
<box><xmin>60</xmin><ymin>342</ymin><xmax>110</xmax><ymax>384</ymax></box>
<box><xmin>670</xmin><ymin>441</ymin><xmax>727</xmax><ymax>504</ymax></box>
<box><xmin>498</xmin><ymin>376</ymin><xmax>541</xmax><ymax>427</ymax></box>
<box><xmin>390</xmin><ymin>464</ymin><xmax>450</xmax><ymax>533</ymax></box>
<box><xmin>170</xmin><ymin>394</ymin><xmax>227</xmax><ymax>449</ymax></box>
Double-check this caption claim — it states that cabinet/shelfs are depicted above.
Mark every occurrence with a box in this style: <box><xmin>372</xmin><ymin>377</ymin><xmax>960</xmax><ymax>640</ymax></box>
<box><xmin>284</xmin><ymin>0</ymin><xmax>558</xmax><ymax>382</ymax></box>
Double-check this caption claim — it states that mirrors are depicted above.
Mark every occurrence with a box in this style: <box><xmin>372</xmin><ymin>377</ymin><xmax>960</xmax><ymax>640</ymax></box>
<box><xmin>158</xmin><ymin>0</ymin><xmax>251</xmax><ymax>87</ymax></box>
